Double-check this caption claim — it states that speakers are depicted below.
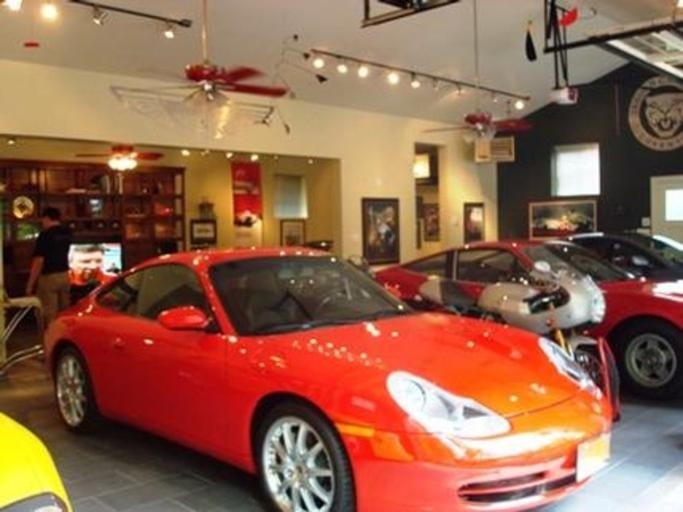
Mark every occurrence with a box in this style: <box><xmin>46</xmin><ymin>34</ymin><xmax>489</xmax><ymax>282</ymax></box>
<box><xmin>476</xmin><ymin>136</ymin><xmax>515</xmax><ymax>163</ymax></box>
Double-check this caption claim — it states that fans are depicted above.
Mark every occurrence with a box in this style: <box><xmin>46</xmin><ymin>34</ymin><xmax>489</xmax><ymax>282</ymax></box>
<box><xmin>74</xmin><ymin>144</ymin><xmax>163</xmax><ymax>162</ymax></box>
<box><xmin>111</xmin><ymin>0</ymin><xmax>288</xmax><ymax>105</ymax></box>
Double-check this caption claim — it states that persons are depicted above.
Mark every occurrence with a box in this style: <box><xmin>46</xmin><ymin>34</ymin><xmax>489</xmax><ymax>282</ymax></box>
<box><xmin>25</xmin><ymin>207</ymin><xmax>71</xmax><ymax>326</ymax></box>
<box><xmin>69</xmin><ymin>244</ymin><xmax>117</xmax><ymax>288</ymax></box>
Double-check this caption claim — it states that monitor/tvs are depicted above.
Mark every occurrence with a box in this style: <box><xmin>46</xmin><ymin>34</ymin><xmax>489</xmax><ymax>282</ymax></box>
<box><xmin>67</xmin><ymin>235</ymin><xmax>126</xmax><ymax>274</ymax></box>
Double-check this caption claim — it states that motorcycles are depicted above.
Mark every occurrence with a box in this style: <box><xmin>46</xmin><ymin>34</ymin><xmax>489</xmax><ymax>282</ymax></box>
<box><xmin>382</xmin><ymin>244</ymin><xmax>624</xmax><ymax>420</ymax></box>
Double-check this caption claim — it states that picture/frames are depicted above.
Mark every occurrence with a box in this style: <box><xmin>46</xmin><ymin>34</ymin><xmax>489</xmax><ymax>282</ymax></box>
<box><xmin>280</xmin><ymin>219</ymin><xmax>305</xmax><ymax>245</ymax></box>
<box><xmin>191</xmin><ymin>219</ymin><xmax>217</xmax><ymax>242</ymax></box>
<box><xmin>361</xmin><ymin>198</ymin><xmax>401</xmax><ymax>264</ymax></box>
<box><xmin>464</xmin><ymin>203</ymin><xmax>484</xmax><ymax>241</ymax></box>
<box><xmin>530</xmin><ymin>200</ymin><xmax>596</xmax><ymax>240</ymax></box>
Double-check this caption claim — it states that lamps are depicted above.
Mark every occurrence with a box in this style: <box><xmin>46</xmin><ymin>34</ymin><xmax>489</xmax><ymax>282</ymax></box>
<box><xmin>186</xmin><ymin>85</ymin><xmax>234</xmax><ymax>103</ymax></box>
<box><xmin>107</xmin><ymin>158</ymin><xmax>138</xmax><ymax>171</ymax></box>
<box><xmin>0</xmin><ymin>0</ymin><xmax>192</xmax><ymax>38</ymax></box>
<box><xmin>311</xmin><ymin>49</ymin><xmax>530</xmax><ymax>109</ymax></box>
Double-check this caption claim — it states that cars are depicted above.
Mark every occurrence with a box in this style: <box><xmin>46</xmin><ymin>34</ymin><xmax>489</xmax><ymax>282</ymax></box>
<box><xmin>531</xmin><ymin>206</ymin><xmax>593</xmax><ymax>233</ymax></box>
<box><xmin>2</xmin><ymin>409</ymin><xmax>77</xmax><ymax>511</ymax></box>
<box><xmin>561</xmin><ymin>220</ymin><xmax>683</xmax><ymax>286</ymax></box>
<box><xmin>370</xmin><ymin>226</ymin><xmax>683</xmax><ymax>405</ymax></box>
<box><xmin>39</xmin><ymin>247</ymin><xmax>625</xmax><ymax>512</ymax></box>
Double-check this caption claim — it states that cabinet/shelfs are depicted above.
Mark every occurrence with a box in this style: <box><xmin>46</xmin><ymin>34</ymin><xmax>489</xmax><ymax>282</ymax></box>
<box><xmin>0</xmin><ymin>157</ymin><xmax>185</xmax><ymax>298</ymax></box>
